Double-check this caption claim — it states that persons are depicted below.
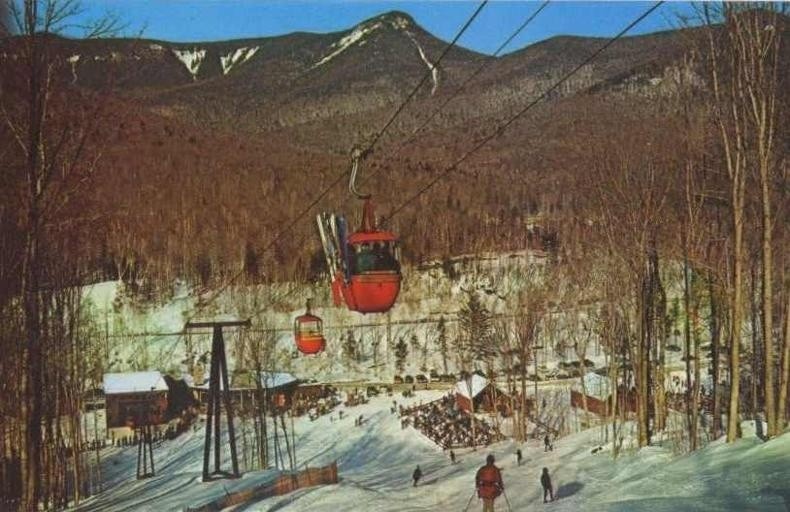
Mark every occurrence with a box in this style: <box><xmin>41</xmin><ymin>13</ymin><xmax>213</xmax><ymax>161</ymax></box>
<box><xmin>390</xmin><ymin>394</ymin><xmax>494</xmax><ymax>454</ymax></box>
<box><xmin>402</xmin><ymin>385</ymin><xmax>416</xmax><ymax>396</ymax></box>
<box><xmin>412</xmin><ymin>464</ymin><xmax>423</xmax><ymax>486</ymax></box>
<box><xmin>449</xmin><ymin>450</ymin><xmax>457</xmax><ymax>465</ymax></box>
<box><xmin>541</xmin><ymin>467</ymin><xmax>554</xmax><ymax>503</ymax></box>
<box><xmin>512</xmin><ymin>449</ymin><xmax>522</xmax><ymax>466</ymax></box>
<box><xmin>543</xmin><ymin>434</ymin><xmax>552</xmax><ymax>452</ymax></box>
<box><xmin>476</xmin><ymin>454</ymin><xmax>503</xmax><ymax>512</ymax></box>
<box><xmin>268</xmin><ymin>385</ymin><xmax>365</xmax><ymax>426</ymax></box>
